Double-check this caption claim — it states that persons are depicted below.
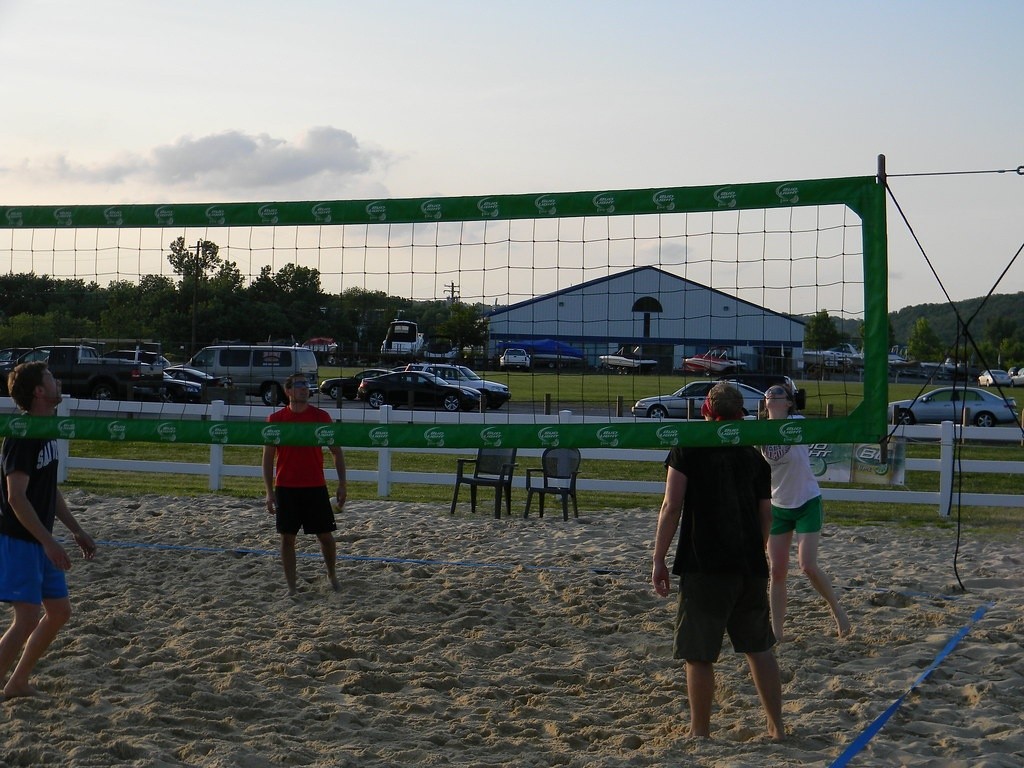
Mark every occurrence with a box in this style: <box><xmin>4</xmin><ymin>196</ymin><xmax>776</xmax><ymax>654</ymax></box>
<box><xmin>748</xmin><ymin>385</ymin><xmax>850</xmax><ymax>642</ymax></box>
<box><xmin>1</xmin><ymin>364</ymin><xmax>97</xmax><ymax>699</ymax></box>
<box><xmin>653</xmin><ymin>384</ymin><xmax>786</xmax><ymax>741</ymax></box>
<box><xmin>262</xmin><ymin>374</ymin><xmax>347</xmax><ymax>598</ymax></box>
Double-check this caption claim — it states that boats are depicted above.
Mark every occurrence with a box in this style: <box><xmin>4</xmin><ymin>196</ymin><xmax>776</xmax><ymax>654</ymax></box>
<box><xmin>682</xmin><ymin>348</ymin><xmax>747</xmax><ymax>374</ymax></box>
<box><xmin>599</xmin><ymin>347</ymin><xmax>659</xmax><ymax>368</ymax></box>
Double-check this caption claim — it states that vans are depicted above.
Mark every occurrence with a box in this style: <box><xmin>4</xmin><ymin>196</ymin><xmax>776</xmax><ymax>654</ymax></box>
<box><xmin>184</xmin><ymin>345</ymin><xmax>320</xmax><ymax>406</ymax></box>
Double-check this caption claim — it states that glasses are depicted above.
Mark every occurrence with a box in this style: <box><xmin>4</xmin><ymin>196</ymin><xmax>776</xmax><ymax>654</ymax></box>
<box><xmin>764</xmin><ymin>386</ymin><xmax>790</xmax><ymax>400</ymax></box>
<box><xmin>291</xmin><ymin>381</ymin><xmax>311</xmax><ymax>389</ymax></box>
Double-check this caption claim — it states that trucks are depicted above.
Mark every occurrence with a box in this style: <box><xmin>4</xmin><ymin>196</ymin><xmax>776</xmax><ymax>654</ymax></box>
<box><xmin>380</xmin><ymin>320</ymin><xmax>425</xmax><ymax>362</ymax></box>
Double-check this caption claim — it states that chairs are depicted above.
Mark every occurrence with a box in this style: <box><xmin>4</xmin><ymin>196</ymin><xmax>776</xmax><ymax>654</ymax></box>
<box><xmin>450</xmin><ymin>446</ymin><xmax>519</xmax><ymax>519</ymax></box>
<box><xmin>448</xmin><ymin>372</ymin><xmax>452</xmax><ymax>377</ymax></box>
<box><xmin>440</xmin><ymin>372</ymin><xmax>446</xmax><ymax>380</ymax></box>
<box><xmin>524</xmin><ymin>447</ymin><xmax>581</xmax><ymax>522</ymax></box>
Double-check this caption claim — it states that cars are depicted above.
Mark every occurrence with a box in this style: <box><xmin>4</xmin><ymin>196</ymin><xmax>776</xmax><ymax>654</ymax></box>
<box><xmin>499</xmin><ymin>348</ymin><xmax>532</xmax><ymax>370</ymax></box>
<box><xmin>887</xmin><ymin>386</ymin><xmax>1019</xmax><ymax>428</ymax></box>
<box><xmin>319</xmin><ymin>366</ymin><xmax>481</xmax><ymax>412</ymax></box>
<box><xmin>102</xmin><ymin>350</ymin><xmax>233</xmax><ymax>404</ymax></box>
<box><xmin>979</xmin><ymin>366</ymin><xmax>1024</xmax><ymax>388</ymax></box>
<box><xmin>632</xmin><ymin>381</ymin><xmax>767</xmax><ymax>419</ymax></box>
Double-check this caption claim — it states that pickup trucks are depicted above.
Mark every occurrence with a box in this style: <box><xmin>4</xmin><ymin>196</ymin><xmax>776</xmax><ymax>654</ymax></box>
<box><xmin>0</xmin><ymin>346</ymin><xmax>142</xmax><ymax>401</ymax></box>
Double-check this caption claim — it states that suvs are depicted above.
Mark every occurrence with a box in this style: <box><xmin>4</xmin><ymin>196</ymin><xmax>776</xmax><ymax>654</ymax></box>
<box><xmin>713</xmin><ymin>373</ymin><xmax>806</xmax><ymax>415</ymax></box>
<box><xmin>404</xmin><ymin>360</ymin><xmax>512</xmax><ymax>410</ymax></box>
